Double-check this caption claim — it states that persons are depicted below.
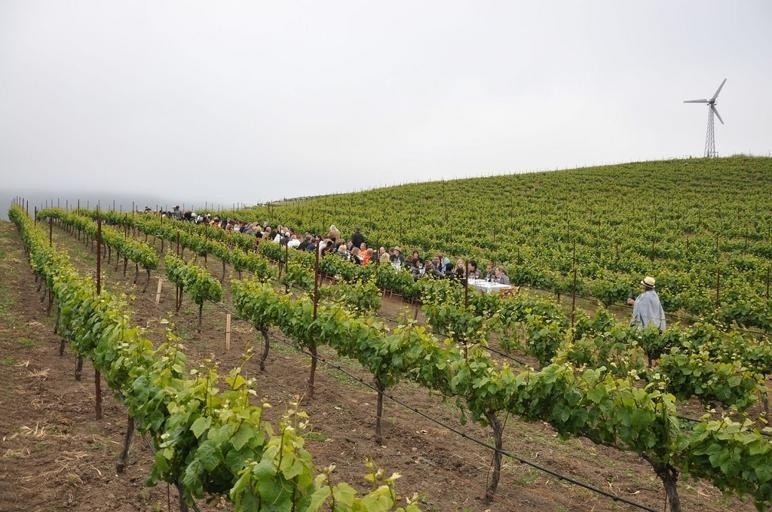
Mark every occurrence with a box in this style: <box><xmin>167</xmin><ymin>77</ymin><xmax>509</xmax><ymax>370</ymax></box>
<box><xmin>628</xmin><ymin>276</ymin><xmax>666</xmax><ymax>332</ymax></box>
<box><xmin>158</xmin><ymin>207</ymin><xmax>511</xmax><ymax>290</ymax></box>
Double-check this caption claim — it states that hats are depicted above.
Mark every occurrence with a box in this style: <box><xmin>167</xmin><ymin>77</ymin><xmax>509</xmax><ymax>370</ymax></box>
<box><xmin>641</xmin><ymin>277</ymin><xmax>656</xmax><ymax>288</ymax></box>
